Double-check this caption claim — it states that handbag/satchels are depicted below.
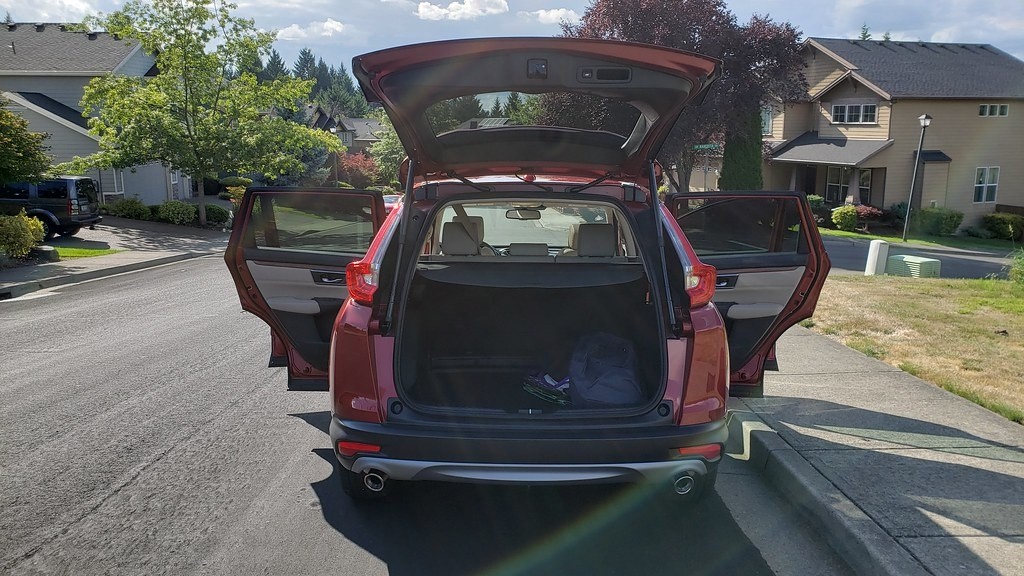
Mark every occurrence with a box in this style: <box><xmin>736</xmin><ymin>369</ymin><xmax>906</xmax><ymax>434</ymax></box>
<box><xmin>519</xmin><ymin>366</ymin><xmax>575</xmax><ymax>408</ymax></box>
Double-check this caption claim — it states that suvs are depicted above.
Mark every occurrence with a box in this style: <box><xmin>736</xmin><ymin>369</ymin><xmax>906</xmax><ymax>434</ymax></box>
<box><xmin>223</xmin><ymin>35</ymin><xmax>832</xmax><ymax>501</ymax></box>
<box><xmin>0</xmin><ymin>175</ymin><xmax>103</xmax><ymax>242</ymax></box>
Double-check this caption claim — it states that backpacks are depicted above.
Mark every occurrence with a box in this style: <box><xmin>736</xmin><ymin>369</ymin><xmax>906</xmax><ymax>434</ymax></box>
<box><xmin>540</xmin><ymin>332</ymin><xmax>653</xmax><ymax>410</ymax></box>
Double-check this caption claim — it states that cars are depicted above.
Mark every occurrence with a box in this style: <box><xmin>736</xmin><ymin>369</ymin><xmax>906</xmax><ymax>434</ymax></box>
<box><xmin>382</xmin><ymin>195</ymin><xmax>402</xmax><ymax>218</ymax></box>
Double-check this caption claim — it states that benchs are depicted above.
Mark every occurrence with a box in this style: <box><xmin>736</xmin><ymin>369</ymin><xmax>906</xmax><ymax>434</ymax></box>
<box><xmin>441</xmin><ymin>221</ymin><xmax>614</xmax><ymax>257</ymax></box>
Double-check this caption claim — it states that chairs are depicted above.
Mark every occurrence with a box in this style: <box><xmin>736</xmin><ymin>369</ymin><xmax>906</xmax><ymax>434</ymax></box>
<box><xmin>557</xmin><ymin>224</ymin><xmax>583</xmax><ymax>255</ymax></box>
<box><xmin>452</xmin><ymin>217</ymin><xmax>485</xmax><ymax>249</ymax></box>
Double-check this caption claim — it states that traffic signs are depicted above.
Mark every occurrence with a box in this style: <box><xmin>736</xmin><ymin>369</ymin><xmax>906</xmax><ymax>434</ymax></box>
<box><xmin>690</xmin><ymin>144</ymin><xmax>720</xmax><ymax>149</ymax></box>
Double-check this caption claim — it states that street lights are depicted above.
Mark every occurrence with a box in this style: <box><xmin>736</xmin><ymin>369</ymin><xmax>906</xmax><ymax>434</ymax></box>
<box><xmin>714</xmin><ymin>170</ymin><xmax>719</xmax><ymax>189</ymax></box>
<box><xmin>330</xmin><ymin>123</ymin><xmax>338</xmax><ymax>188</ymax></box>
<box><xmin>902</xmin><ymin>114</ymin><xmax>933</xmax><ymax>241</ymax></box>
